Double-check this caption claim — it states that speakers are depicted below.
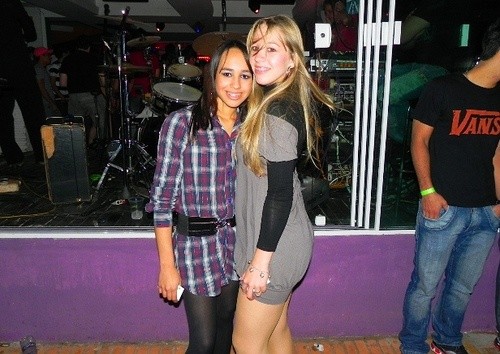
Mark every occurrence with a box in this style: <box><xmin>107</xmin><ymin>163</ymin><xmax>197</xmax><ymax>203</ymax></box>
<box><xmin>315</xmin><ymin>23</ymin><xmax>332</xmax><ymax>49</ymax></box>
<box><xmin>41</xmin><ymin>123</ymin><xmax>92</xmax><ymax>206</ymax></box>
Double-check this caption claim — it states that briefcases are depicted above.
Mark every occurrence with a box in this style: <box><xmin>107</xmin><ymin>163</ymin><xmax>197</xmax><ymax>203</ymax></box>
<box><xmin>41</xmin><ymin>115</ymin><xmax>92</xmax><ymax>205</ymax></box>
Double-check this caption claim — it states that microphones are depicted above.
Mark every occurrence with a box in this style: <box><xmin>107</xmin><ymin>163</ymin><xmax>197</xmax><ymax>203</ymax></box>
<box><xmin>119</xmin><ymin>7</ymin><xmax>131</xmax><ymax>29</ymax></box>
<box><xmin>177</xmin><ymin>44</ymin><xmax>184</xmax><ymax>64</ymax></box>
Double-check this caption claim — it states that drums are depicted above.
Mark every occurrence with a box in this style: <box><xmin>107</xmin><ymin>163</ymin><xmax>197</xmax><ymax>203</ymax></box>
<box><xmin>165</xmin><ymin>63</ymin><xmax>203</xmax><ymax>90</ymax></box>
<box><xmin>117</xmin><ymin>118</ymin><xmax>150</xmax><ymax>146</ymax></box>
<box><xmin>147</xmin><ymin>81</ymin><xmax>202</xmax><ymax>117</ymax></box>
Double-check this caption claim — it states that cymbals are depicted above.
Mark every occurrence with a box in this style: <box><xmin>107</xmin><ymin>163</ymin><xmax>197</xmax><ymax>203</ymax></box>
<box><xmin>192</xmin><ymin>31</ymin><xmax>247</xmax><ymax>57</ymax></box>
<box><xmin>96</xmin><ymin>61</ymin><xmax>151</xmax><ymax>75</ymax></box>
<box><xmin>125</xmin><ymin>36</ymin><xmax>163</xmax><ymax>48</ymax></box>
<box><xmin>90</xmin><ymin>12</ymin><xmax>154</xmax><ymax>30</ymax></box>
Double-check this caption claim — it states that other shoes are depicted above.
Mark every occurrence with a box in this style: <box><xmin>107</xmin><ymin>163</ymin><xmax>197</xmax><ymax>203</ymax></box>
<box><xmin>34</xmin><ymin>151</ymin><xmax>46</xmax><ymax>165</ymax></box>
<box><xmin>3</xmin><ymin>141</ymin><xmax>26</xmax><ymax>166</ymax></box>
<box><xmin>430</xmin><ymin>338</ymin><xmax>469</xmax><ymax>354</ymax></box>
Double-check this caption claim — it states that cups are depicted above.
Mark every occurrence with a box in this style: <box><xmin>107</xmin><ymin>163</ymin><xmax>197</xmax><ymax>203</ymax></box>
<box><xmin>128</xmin><ymin>197</ymin><xmax>146</xmax><ymax>220</ymax></box>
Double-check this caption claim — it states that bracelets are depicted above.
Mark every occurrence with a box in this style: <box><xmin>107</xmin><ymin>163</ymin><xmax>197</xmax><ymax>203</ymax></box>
<box><xmin>248</xmin><ymin>260</ymin><xmax>271</xmax><ymax>279</ymax></box>
<box><xmin>419</xmin><ymin>186</ymin><xmax>437</xmax><ymax>197</ymax></box>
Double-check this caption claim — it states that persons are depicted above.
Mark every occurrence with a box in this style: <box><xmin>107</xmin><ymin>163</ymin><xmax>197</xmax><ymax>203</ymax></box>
<box><xmin>181</xmin><ymin>46</ymin><xmax>201</xmax><ymax>86</ymax></box>
<box><xmin>146</xmin><ymin>39</ymin><xmax>254</xmax><ymax>354</ymax></box>
<box><xmin>59</xmin><ymin>35</ymin><xmax>100</xmax><ymax>149</ymax></box>
<box><xmin>231</xmin><ymin>16</ymin><xmax>334</xmax><ymax>354</ymax></box>
<box><xmin>111</xmin><ymin>27</ymin><xmax>160</xmax><ymax>110</ymax></box>
<box><xmin>301</xmin><ymin>0</ymin><xmax>359</xmax><ymax>59</ymax></box>
<box><xmin>0</xmin><ymin>0</ymin><xmax>48</xmax><ymax>167</ymax></box>
<box><xmin>397</xmin><ymin>20</ymin><xmax>500</xmax><ymax>354</ymax></box>
<box><xmin>32</xmin><ymin>46</ymin><xmax>69</xmax><ymax>123</ymax></box>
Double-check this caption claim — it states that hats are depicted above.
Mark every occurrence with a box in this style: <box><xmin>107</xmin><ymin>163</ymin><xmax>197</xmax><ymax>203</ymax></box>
<box><xmin>34</xmin><ymin>47</ymin><xmax>54</xmax><ymax>57</ymax></box>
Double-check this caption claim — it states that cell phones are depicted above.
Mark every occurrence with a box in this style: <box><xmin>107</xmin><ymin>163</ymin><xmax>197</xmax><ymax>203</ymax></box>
<box><xmin>177</xmin><ymin>286</ymin><xmax>184</xmax><ymax>301</ymax></box>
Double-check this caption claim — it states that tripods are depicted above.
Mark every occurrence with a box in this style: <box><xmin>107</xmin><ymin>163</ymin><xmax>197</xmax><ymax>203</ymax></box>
<box><xmin>89</xmin><ymin>29</ymin><xmax>156</xmax><ymax>203</ymax></box>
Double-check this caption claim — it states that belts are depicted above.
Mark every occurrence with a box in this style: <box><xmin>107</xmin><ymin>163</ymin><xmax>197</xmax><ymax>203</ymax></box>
<box><xmin>173</xmin><ymin>210</ymin><xmax>237</xmax><ymax>238</ymax></box>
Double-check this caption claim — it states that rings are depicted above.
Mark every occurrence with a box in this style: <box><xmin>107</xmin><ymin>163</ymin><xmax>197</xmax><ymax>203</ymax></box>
<box><xmin>250</xmin><ymin>288</ymin><xmax>256</xmax><ymax>291</ymax></box>
